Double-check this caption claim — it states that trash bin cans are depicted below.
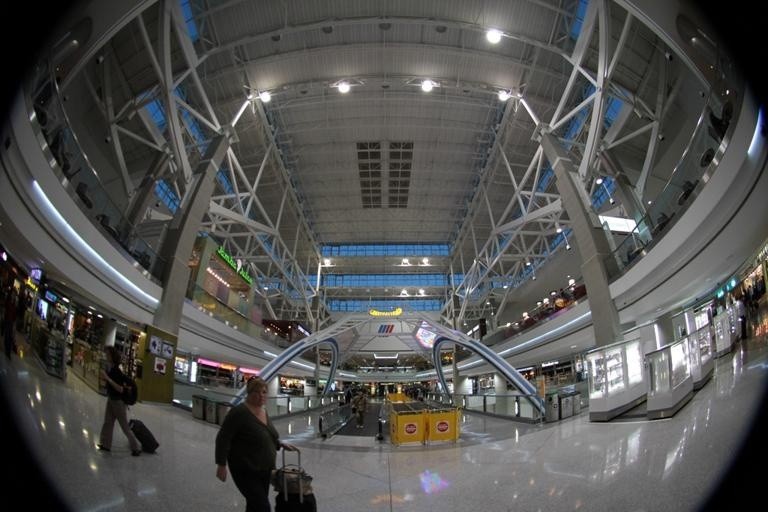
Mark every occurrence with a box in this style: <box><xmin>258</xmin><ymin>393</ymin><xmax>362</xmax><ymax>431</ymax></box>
<box><xmin>544</xmin><ymin>391</ymin><xmax>581</xmax><ymax>423</ymax></box>
<box><xmin>191</xmin><ymin>394</ymin><xmax>234</xmax><ymax>426</ymax></box>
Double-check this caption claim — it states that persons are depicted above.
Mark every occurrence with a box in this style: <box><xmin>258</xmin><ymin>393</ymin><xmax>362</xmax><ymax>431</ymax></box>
<box><xmin>0</xmin><ymin>283</ymin><xmax>105</xmax><ymax>366</ymax></box>
<box><xmin>706</xmin><ymin>275</ymin><xmax>767</xmax><ymax>340</ymax></box>
<box><xmin>96</xmin><ymin>345</ymin><xmax>144</xmax><ymax>459</ymax></box>
<box><xmin>350</xmin><ymin>390</ymin><xmax>369</xmax><ymax>431</ymax></box>
<box><xmin>281</xmin><ymin>380</ymin><xmax>434</xmax><ymax>405</ymax></box>
<box><xmin>215</xmin><ymin>374</ymin><xmax>298</xmax><ymax>511</ymax></box>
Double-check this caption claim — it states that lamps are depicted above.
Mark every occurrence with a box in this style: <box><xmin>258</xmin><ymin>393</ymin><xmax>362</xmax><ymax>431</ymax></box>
<box><xmin>531</xmin><ymin>265</ymin><xmax>536</xmax><ymax>281</ymax></box>
<box><xmin>553</xmin><ymin>213</ymin><xmax>562</xmax><ymax>234</ymax></box>
<box><xmin>486</xmin><ymin>297</ymin><xmax>491</xmax><ymax>305</ymax></box>
<box><xmin>105</xmin><ymin>129</ymin><xmax>113</xmax><ymax>145</ymax></box>
<box><xmin>502</xmin><ymin>280</ymin><xmax>508</xmax><ymax>289</ymax></box>
<box><xmin>155</xmin><ymin>166</ymin><xmax>181</xmax><ymax>207</ymax></box>
<box><xmin>603</xmin><ymin>182</ymin><xmax>615</xmax><ymax>205</ymax></box>
<box><xmin>63</xmin><ymin>91</ymin><xmax>71</xmax><ymax>103</ymax></box>
<box><xmin>490</xmin><ymin>305</ymin><xmax>494</xmax><ymax>316</ymax></box>
<box><xmin>595</xmin><ymin>174</ymin><xmax>602</xmax><ymax>185</ymax></box>
<box><xmin>97</xmin><ymin>48</ymin><xmax>113</xmax><ymax>65</ymax></box>
<box><xmin>525</xmin><ymin>256</ymin><xmax>531</xmax><ymax>267</ymax></box>
<box><xmin>562</xmin><ymin>230</ymin><xmax>571</xmax><ymax>251</ymax></box>
<box><xmin>463</xmin><ymin>314</ymin><xmax>468</xmax><ymax>326</ymax></box>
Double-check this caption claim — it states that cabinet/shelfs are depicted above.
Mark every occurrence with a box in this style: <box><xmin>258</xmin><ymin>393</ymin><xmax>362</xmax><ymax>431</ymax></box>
<box><xmin>21</xmin><ymin>309</ymin><xmax>139</xmax><ymax>397</ymax></box>
<box><xmin>584</xmin><ymin>303</ymin><xmax>741</xmax><ymax>422</ymax></box>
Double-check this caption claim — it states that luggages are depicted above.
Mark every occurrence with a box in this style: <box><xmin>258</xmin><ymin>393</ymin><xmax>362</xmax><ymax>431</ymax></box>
<box><xmin>127</xmin><ymin>408</ymin><xmax>158</xmax><ymax>455</ymax></box>
<box><xmin>276</xmin><ymin>448</ymin><xmax>318</xmax><ymax>512</ymax></box>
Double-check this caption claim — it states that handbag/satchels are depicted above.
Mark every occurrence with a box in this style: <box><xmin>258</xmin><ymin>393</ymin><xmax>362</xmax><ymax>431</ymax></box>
<box><xmin>274</xmin><ymin>464</ymin><xmax>313</xmax><ymax>496</ymax></box>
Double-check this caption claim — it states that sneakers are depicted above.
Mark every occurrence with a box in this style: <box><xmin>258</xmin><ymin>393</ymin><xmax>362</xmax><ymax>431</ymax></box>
<box><xmin>131</xmin><ymin>450</ymin><xmax>141</xmax><ymax>457</ymax></box>
<box><xmin>97</xmin><ymin>444</ymin><xmax>110</xmax><ymax>452</ymax></box>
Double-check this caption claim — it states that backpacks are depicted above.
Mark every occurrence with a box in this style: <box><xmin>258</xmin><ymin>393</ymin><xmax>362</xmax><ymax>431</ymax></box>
<box><xmin>123</xmin><ymin>374</ymin><xmax>137</xmax><ymax>405</ymax></box>
